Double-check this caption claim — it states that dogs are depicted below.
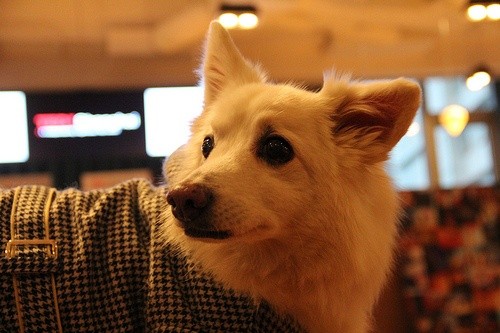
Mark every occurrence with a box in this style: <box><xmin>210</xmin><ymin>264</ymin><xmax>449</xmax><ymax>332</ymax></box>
<box><xmin>156</xmin><ymin>20</ymin><xmax>422</xmax><ymax>333</ymax></box>
